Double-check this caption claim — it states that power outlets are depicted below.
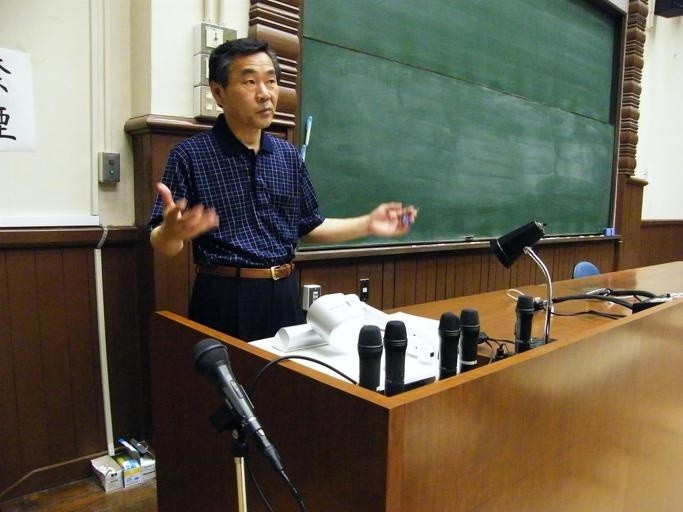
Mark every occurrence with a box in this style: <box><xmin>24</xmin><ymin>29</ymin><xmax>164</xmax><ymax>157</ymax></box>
<box><xmin>301</xmin><ymin>284</ymin><xmax>322</xmax><ymax>311</ymax></box>
<box><xmin>359</xmin><ymin>277</ymin><xmax>369</xmax><ymax>303</ymax></box>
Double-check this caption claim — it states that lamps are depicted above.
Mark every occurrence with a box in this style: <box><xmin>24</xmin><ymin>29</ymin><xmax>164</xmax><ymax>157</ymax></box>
<box><xmin>489</xmin><ymin>220</ymin><xmax>559</xmax><ymax>349</ymax></box>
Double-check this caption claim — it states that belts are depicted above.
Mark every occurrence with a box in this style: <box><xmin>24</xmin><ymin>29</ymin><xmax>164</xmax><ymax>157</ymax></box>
<box><xmin>199</xmin><ymin>263</ymin><xmax>295</xmax><ymax>278</ymax></box>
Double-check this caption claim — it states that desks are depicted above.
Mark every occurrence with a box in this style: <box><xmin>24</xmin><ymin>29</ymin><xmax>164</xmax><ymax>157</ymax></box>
<box><xmin>149</xmin><ymin>259</ymin><xmax>683</xmax><ymax>512</ymax></box>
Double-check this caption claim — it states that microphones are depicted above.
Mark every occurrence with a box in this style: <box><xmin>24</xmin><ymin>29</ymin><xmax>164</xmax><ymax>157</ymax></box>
<box><xmin>460</xmin><ymin>310</ymin><xmax>480</xmax><ymax>373</ymax></box>
<box><xmin>190</xmin><ymin>338</ymin><xmax>284</xmax><ymax>471</ymax></box>
<box><xmin>384</xmin><ymin>321</ymin><xmax>407</xmax><ymax>397</ymax></box>
<box><xmin>515</xmin><ymin>296</ymin><xmax>535</xmax><ymax>353</ymax></box>
<box><xmin>439</xmin><ymin>312</ymin><xmax>461</xmax><ymax>381</ymax></box>
<box><xmin>358</xmin><ymin>326</ymin><xmax>382</xmax><ymax>391</ymax></box>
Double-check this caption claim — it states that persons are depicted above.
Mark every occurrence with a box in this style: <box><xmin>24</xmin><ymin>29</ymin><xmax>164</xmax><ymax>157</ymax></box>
<box><xmin>145</xmin><ymin>37</ymin><xmax>420</xmax><ymax>344</ymax></box>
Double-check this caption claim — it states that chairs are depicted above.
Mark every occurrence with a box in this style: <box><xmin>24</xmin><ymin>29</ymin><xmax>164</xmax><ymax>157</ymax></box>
<box><xmin>572</xmin><ymin>262</ymin><xmax>599</xmax><ymax>278</ymax></box>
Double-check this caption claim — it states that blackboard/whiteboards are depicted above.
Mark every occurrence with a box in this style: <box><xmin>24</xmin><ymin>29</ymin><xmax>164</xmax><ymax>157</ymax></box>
<box><xmin>295</xmin><ymin>0</ymin><xmax>629</xmax><ymax>262</ymax></box>
